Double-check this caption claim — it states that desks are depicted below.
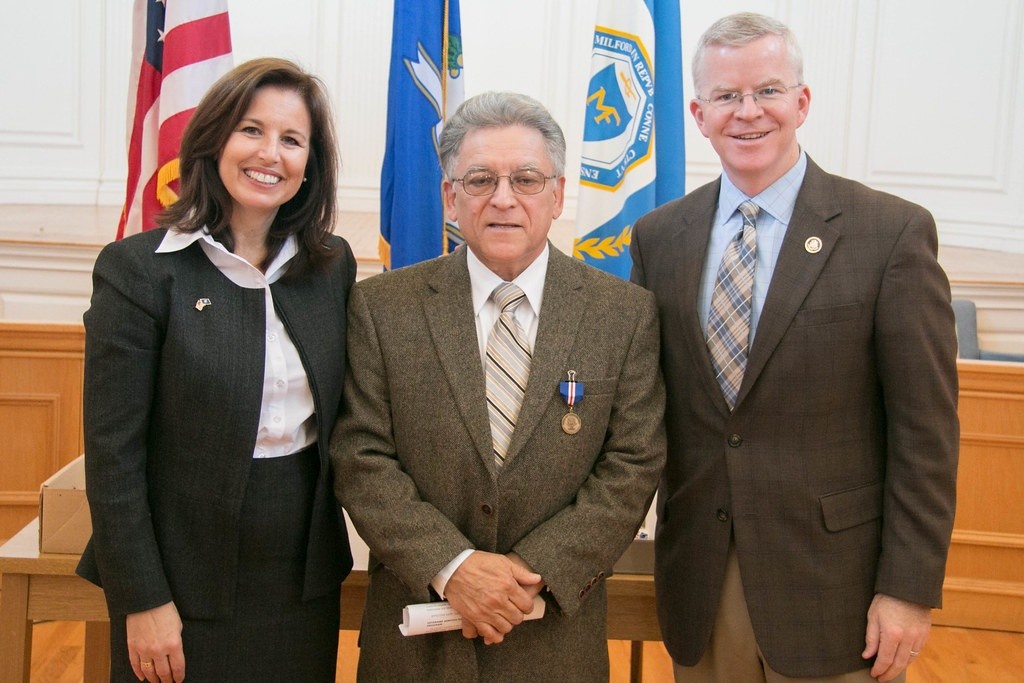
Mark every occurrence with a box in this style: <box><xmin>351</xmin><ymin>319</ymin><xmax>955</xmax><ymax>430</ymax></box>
<box><xmin>0</xmin><ymin>492</ymin><xmax>674</xmax><ymax>683</ymax></box>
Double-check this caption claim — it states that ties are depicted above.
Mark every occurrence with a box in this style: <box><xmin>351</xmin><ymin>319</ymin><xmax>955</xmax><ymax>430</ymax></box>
<box><xmin>705</xmin><ymin>199</ymin><xmax>757</xmax><ymax>409</ymax></box>
<box><xmin>484</xmin><ymin>280</ymin><xmax>532</xmax><ymax>472</ymax></box>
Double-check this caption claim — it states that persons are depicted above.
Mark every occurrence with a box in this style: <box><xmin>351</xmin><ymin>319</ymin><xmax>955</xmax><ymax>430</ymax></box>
<box><xmin>454</xmin><ymin>12</ymin><xmax>960</xmax><ymax>683</ymax></box>
<box><xmin>327</xmin><ymin>94</ymin><xmax>666</xmax><ymax>683</ymax></box>
<box><xmin>76</xmin><ymin>57</ymin><xmax>355</xmax><ymax>683</ymax></box>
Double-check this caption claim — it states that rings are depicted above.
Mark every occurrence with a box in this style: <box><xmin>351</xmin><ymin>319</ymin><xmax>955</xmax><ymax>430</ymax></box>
<box><xmin>140</xmin><ymin>663</ymin><xmax>151</xmax><ymax>667</ymax></box>
<box><xmin>910</xmin><ymin>651</ymin><xmax>918</xmax><ymax>656</ymax></box>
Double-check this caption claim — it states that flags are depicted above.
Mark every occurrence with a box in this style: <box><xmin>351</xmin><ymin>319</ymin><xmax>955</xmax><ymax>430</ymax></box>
<box><xmin>574</xmin><ymin>0</ymin><xmax>686</xmax><ymax>282</ymax></box>
<box><xmin>113</xmin><ymin>0</ymin><xmax>235</xmax><ymax>243</ymax></box>
<box><xmin>379</xmin><ymin>0</ymin><xmax>465</xmax><ymax>272</ymax></box>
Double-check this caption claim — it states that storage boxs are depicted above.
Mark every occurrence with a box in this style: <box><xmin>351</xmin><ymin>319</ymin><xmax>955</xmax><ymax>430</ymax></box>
<box><xmin>38</xmin><ymin>454</ymin><xmax>96</xmax><ymax>554</ymax></box>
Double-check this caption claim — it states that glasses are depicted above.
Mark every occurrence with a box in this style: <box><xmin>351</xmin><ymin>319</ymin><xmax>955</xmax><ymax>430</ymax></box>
<box><xmin>697</xmin><ymin>84</ymin><xmax>803</xmax><ymax>109</ymax></box>
<box><xmin>450</xmin><ymin>171</ymin><xmax>557</xmax><ymax>197</ymax></box>
